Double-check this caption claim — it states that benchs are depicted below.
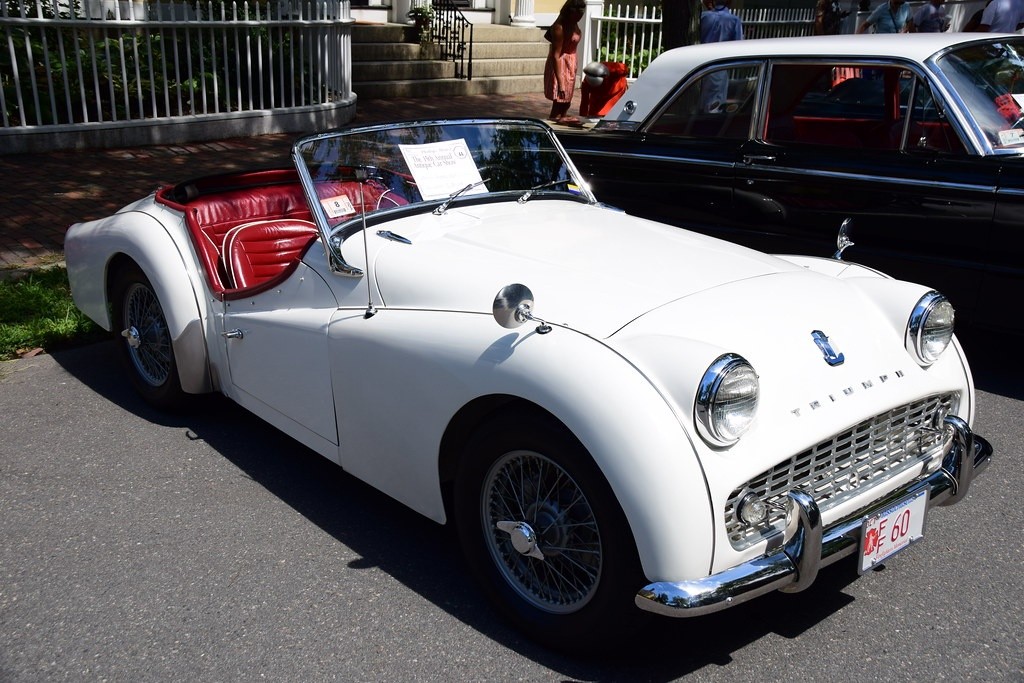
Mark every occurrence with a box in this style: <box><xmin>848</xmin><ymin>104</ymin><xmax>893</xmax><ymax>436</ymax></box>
<box><xmin>188</xmin><ymin>178</ymin><xmax>410</xmax><ymax>289</ymax></box>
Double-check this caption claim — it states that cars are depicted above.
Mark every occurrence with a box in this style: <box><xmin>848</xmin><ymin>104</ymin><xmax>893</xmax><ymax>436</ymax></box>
<box><xmin>433</xmin><ymin>32</ymin><xmax>1024</xmax><ymax>341</ymax></box>
<box><xmin>64</xmin><ymin>117</ymin><xmax>993</xmax><ymax>632</ymax></box>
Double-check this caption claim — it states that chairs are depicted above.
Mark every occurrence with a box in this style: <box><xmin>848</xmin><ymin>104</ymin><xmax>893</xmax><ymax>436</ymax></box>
<box><xmin>222</xmin><ymin>218</ymin><xmax>319</xmax><ymax>290</ymax></box>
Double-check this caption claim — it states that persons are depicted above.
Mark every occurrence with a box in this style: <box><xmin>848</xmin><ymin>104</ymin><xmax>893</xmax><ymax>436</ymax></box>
<box><xmin>909</xmin><ymin>0</ymin><xmax>950</xmax><ymax>32</ymax></box>
<box><xmin>815</xmin><ymin>0</ymin><xmax>852</xmax><ymax>35</ymax></box>
<box><xmin>543</xmin><ymin>0</ymin><xmax>586</xmax><ymax>118</ymax></box>
<box><xmin>857</xmin><ymin>0</ymin><xmax>914</xmax><ymax>33</ymax></box>
<box><xmin>978</xmin><ymin>0</ymin><xmax>1024</xmax><ymax>60</ymax></box>
<box><xmin>700</xmin><ymin>0</ymin><xmax>743</xmax><ymax>113</ymax></box>
